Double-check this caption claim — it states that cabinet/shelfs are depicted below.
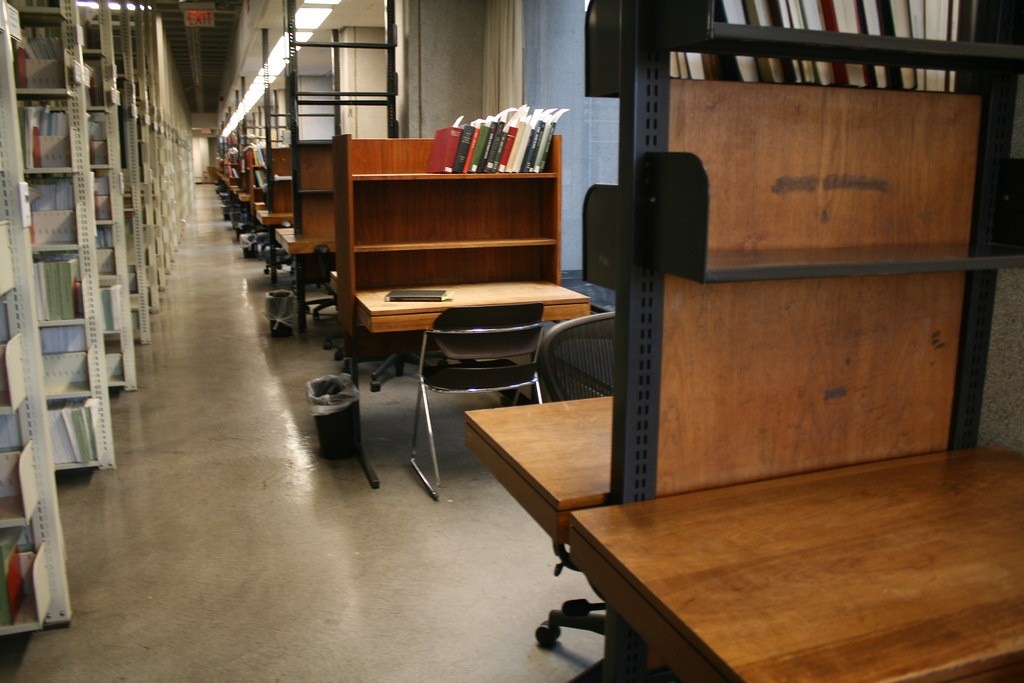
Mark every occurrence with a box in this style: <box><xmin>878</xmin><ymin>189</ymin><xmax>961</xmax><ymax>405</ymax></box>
<box><xmin>0</xmin><ymin>0</ymin><xmax>1024</xmax><ymax>683</ymax></box>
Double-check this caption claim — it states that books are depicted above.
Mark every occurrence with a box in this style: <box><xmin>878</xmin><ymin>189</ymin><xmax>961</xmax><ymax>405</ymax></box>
<box><xmin>0</xmin><ymin>0</ymin><xmax>157</xmax><ymax>627</ymax></box>
<box><xmin>668</xmin><ymin>0</ymin><xmax>962</xmax><ymax>92</ymax></box>
<box><xmin>428</xmin><ymin>105</ymin><xmax>570</xmax><ymax>174</ymax></box>
<box><xmin>253</xmin><ymin>148</ymin><xmax>264</xmax><ymax>166</ymax></box>
<box><xmin>384</xmin><ymin>290</ymin><xmax>454</xmax><ymax>302</ymax></box>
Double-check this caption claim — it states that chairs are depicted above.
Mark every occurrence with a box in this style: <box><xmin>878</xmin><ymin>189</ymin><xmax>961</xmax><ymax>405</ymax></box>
<box><xmin>409</xmin><ymin>303</ymin><xmax>544</xmax><ymax>501</ymax></box>
<box><xmin>534</xmin><ymin>309</ymin><xmax>640</xmax><ymax>683</ymax></box>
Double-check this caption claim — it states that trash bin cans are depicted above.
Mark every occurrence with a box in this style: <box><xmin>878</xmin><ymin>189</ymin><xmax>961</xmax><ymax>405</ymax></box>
<box><xmin>214</xmin><ymin>181</ymin><xmax>259</xmax><ymax>259</ymax></box>
<box><xmin>307</xmin><ymin>376</ymin><xmax>361</xmax><ymax>459</ymax></box>
<box><xmin>265</xmin><ymin>290</ymin><xmax>294</xmax><ymax>337</ymax></box>
